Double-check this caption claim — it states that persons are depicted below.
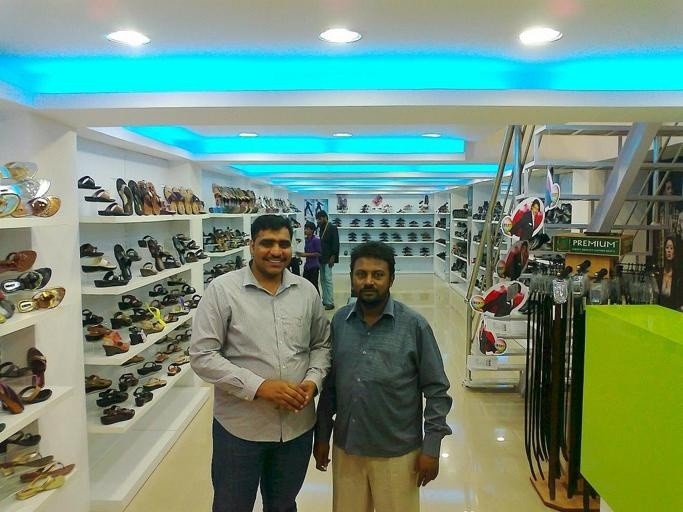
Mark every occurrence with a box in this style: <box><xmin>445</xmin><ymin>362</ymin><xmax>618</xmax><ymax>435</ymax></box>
<box><xmin>315</xmin><ymin>199</ymin><xmax>324</xmax><ymax>217</ymax></box>
<box><xmin>310</xmin><ymin>239</ymin><xmax>452</xmax><ymax>512</ymax></box>
<box><xmin>659</xmin><ymin>177</ymin><xmax>676</xmax><ymax>197</ymax></box>
<box><xmin>478</xmin><ymin>320</ymin><xmax>498</xmax><ymax>355</ymax></box>
<box><xmin>294</xmin><ymin>221</ymin><xmax>322</xmax><ymax>302</ymax></box>
<box><xmin>502</xmin><ymin>241</ymin><xmax>528</xmax><ymax>281</ymax></box>
<box><xmin>304</xmin><ymin>199</ymin><xmax>314</xmax><ymax>219</ymax></box>
<box><xmin>477</xmin><ymin>281</ymin><xmax>520</xmax><ymax>318</ymax></box>
<box><xmin>507</xmin><ymin>200</ymin><xmax>541</xmax><ymax>243</ymax></box>
<box><xmin>188</xmin><ymin>213</ymin><xmax>332</xmax><ymax>512</ymax></box>
<box><xmin>656</xmin><ymin>233</ymin><xmax>681</xmax><ymax>311</ymax></box>
<box><xmin>314</xmin><ymin>210</ymin><xmax>339</xmax><ymax>311</ymax></box>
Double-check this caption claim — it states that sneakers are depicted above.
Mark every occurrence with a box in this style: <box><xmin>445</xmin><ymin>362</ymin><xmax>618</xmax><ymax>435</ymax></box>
<box><xmin>324</xmin><ymin>305</ymin><xmax>335</xmax><ymax>310</ymax></box>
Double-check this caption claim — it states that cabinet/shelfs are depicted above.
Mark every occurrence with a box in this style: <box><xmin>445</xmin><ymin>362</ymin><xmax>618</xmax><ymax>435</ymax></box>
<box><xmin>433</xmin><ymin>168</ymin><xmax>584</xmax><ymax>303</ymax></box>
<box><xmin>326</xmin><ymin>211</ymin><xmax>433</xmax><ymax>275</ymax></box>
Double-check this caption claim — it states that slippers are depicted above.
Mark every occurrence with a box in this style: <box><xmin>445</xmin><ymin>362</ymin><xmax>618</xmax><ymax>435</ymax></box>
<box><xmin>258</xmin><ymin>197</ymin><xmax>302</xmax><ymax>242</ymax></box>
<box><xmin>79</xmin><ymin>235</ymin><xmax>206</xmax><ymax>423</ymax></box>
<box><xmin>1</xmin><ymin>347</ymin><xmax>53</xmax><ymax>413</ymax></box>
<box><xmin>332</xmin><ymin>215</ymin><xmax>431</xmax><ymax>257</ymax></box>
<box><xmin>79</xmin><ymin>174</ymin><xmax>206</xmax><ymax>220</ymax></box>
<box><xmin>0</xmin><ymin>252</ymin><xmax>65</xmax><ymax>324</ymax></box>
<box><xmin>0</xmin><ymin>158</ymin><xmax>62</xmax><ymax>218</ymax></box>
<box><xmin>0</xmin><ymin>432</ymin><xmax>73</xmax><ymax>501</ymax></box>
<box><xmin>203</xmin><ymin>184</ymin><xmax>258</xmax><ymax>285</ymax></box>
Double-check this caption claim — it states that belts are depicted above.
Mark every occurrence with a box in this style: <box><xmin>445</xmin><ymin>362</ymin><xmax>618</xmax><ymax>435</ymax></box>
<box><xmin>524</xmin><ymin>273</ymin><xmax>606</xmax><ymax>511</ymax></box>
